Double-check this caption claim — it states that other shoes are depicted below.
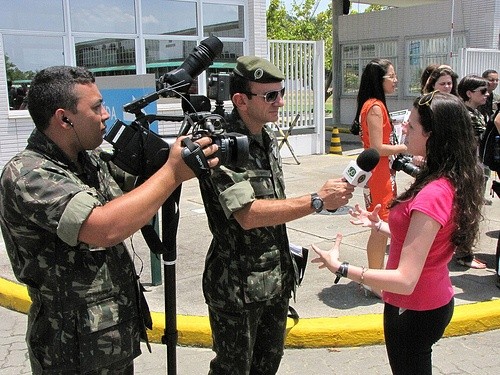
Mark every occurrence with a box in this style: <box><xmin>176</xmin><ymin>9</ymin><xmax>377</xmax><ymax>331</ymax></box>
<box><xmin>456</xmin><ymin>255</ymin><xmax>487</xmax><ymax>269</ymax></box>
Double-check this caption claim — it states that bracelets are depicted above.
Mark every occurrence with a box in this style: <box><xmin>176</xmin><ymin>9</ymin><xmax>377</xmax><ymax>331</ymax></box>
<box><xmin>334</xmin><ymin>262</ymin><xmax>350</xmax><ymax>284</ymax></box>
<box><xmin>377</xmin><ymin>220</ymin><xmax>383</xmax><ymax>232</ymax></box>
<box><xmin>360</xmin><ymin>266</ymin><xmax>365</xmax><ymax>288</ymax></box>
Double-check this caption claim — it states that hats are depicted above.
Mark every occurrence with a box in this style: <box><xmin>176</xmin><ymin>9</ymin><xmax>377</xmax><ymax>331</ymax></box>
<box><xmin>233</xmin><ymin>55</ymin><xmax>285</xmax><ymax>83</ymax></box>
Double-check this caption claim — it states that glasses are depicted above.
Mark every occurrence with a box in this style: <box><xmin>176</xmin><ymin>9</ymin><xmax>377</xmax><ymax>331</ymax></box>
<box><xmin>471</xmin><ymin>88</ymin><xmax>488</xmax><ymax>95</ymax></box>
<box><xmin>244</xmin><ymin>86</ymin><xmax>286</xmax><ymax>104</ymax></box>
<box><xmin>382</xmin><ymin>72</ymin><xmax>399</xmax><ymax>81</ymax></box>
<box><xmin>418</xmin><ymin>89</ymin><xmax>441</xmax><ymax>111</ymax></box>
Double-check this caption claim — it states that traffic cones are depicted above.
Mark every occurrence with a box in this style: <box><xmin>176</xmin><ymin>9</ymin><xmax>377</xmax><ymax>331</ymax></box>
<box><xmin>329</xmin><ymin>126</ymin><xmax>342</xmax><ymax>156</ymax></box>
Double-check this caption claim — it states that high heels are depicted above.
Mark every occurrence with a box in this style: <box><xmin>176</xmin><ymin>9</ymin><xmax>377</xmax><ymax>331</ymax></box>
<box><xmin>361</xmin><ymin>283</ymin><xmax>383</xmax><ymax>299</ymax></box>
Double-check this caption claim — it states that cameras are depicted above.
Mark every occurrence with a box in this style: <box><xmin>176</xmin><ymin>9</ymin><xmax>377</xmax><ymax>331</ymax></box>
<box><xmin>391</xmin><ymin>152</ymin><xmax>422</xmax><ymax>179</ymax></box>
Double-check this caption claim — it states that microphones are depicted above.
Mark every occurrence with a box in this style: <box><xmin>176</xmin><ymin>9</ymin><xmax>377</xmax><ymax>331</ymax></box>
<box><xmin>327</xmin><ymin>148</ymin><xmax>378</xmax><ymax>213</ymax></box>
<box><xmin>179</xmin><ymin>35</ymin><xmax>223</xmax><ymax>78</ymax></box>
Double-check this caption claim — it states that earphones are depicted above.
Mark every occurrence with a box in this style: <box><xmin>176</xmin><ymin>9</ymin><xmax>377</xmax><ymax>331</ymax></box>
<box><xmin>62</xmin><ymin>117</ymin><xmax>69</xmax><ymax>123</ymax></box>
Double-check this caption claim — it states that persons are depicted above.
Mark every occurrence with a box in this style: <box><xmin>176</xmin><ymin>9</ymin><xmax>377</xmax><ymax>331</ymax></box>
<box><xmin>353</xmin><ymin>60</ymin><xmax>408</xmax><ymax>299</ymax></box>
<box><xmin>483</xmin><ymin>69</ymin><xmax>500</xmax><ymax>280</ymax></box>
<box><xmin>459</xmin><ymin>74</ymin><xmax>490</xmax><ymax>270</ymax></box>
<box><xmin>310</xmin><ymin>90</ymin><xmax>485</xmax><ymax>375</ymax></box>
<box><xmin>0</xmin><ymin>66</ymin><xmax>220</xmax><ymax>375</ymax></box>
<box><xmin>199</xmin><ymin>54</ymin><xmax>354</xmax><ymax>375</ymax></box>
<box><xmin>421</xmin><ymin>65</ymin><xmax>459</xmax><ymax>98</ymax></box>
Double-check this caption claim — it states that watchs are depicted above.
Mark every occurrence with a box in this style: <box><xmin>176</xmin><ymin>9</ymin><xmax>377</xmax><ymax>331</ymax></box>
<box><xmin>311</xmin><ymin>193</ymin><xmax>323</xmax><ymax>212</ymax></box>
